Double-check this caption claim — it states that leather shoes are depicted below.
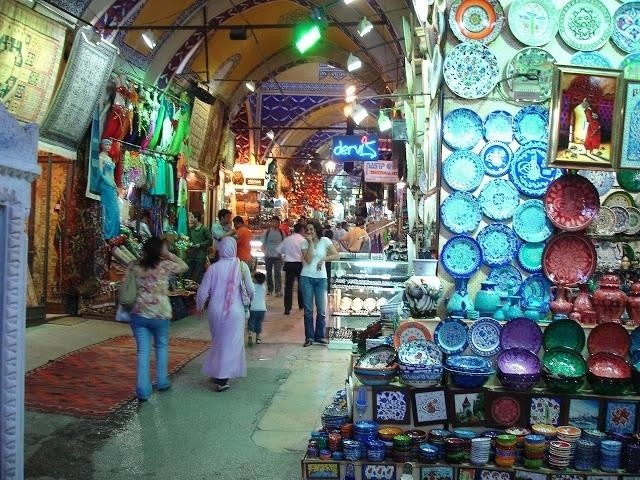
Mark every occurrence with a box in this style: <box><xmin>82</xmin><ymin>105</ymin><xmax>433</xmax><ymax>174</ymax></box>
<box><xmin>299</xmin><ymin>305</ymin><xmax>304</xmax><ymax>309</ymax></box>
<box><xmin>304</xmin><ymin>341</ymin><xmax>312</xmax><ymax>347</ymax></box>
<box><xmin>314</xmin><ymin>338</ymin><xmax>328</xmax><ymax>344</ymax></box>
<box><xmin>284</xmin><ymin>310</ymin><xmax>290</xmax><ymax>315</ymax></box>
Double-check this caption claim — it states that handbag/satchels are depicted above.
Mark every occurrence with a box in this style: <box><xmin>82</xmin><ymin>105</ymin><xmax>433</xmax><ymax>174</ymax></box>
<box><xmin>239</xmin><ymin>276</ymin><xmax>253</xmax><ymax>307</ymax></box>
<box><xmin>118</xmin><ymin>260</ymin><xmax>137</xmax><ymax>311</ymax></box>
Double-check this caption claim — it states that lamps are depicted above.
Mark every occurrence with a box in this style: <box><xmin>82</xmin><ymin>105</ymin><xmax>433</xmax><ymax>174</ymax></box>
<box><xmin>246</xmin><ymin>78</ymin><xmax>256</xmax><ymax>93</ymax></box>
<box><xmin>142</xmin><ymin>27</ymin><xmax>158</xmax><ymax>50</ymax></box>
<box><xmin>266</xmin><ymin>128</ymin><xmax>275</xmax><ymax>141</ymax></box>
<box><xmin>334</xmin><ymin>0</ymin><xmax>406</xmax><ymax>135</ymax></box>
<box><xmin>325</xmin><ymin>146</ymin><xmax>338</xmax><ymax>175</ymax></box>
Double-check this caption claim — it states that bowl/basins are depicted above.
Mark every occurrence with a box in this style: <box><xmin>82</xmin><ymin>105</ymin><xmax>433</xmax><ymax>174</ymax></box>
<box><xmin>348</xmin><ymin>365</ymin><xmax>638</xmax><ymax>396</ymax></box>
<box><xmin>305</xmin><ymin>398</ymin><xmax>639</xmax><ymax>473</ymax></box>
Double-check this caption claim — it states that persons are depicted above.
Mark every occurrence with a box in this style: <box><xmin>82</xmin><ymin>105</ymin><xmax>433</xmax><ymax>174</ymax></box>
<box><xmin>261</xmin><ymin>215</ymin><xmax>369</xmax><ymax>315</ymax></box>
<box><xmin>127</xmin><ymin>237</ymin><xmax>189</xmax><ymax>402</ymax></box>
<box><xmin>99</xmin><ymin>77</ymin><xmax>188</xmax><ymax>239</ymax></box>
<box><xmin>192</xmin><ymin>236</ymin><xmax>255</xmax><ymax>393</ymax></box>
<box><xmin>300</xmin><ymin>218</ymin><xmax>340</xmax><ymax>347</ymax></box>
<box><xmin>182</xmin><ymin>211</ymin><xmax>254</xmax><ymax>285</ymax></box>
<box><xmin>248</xmin><ymin>272</ymin><xmax>267</xmax><ymax>346</ymax></box>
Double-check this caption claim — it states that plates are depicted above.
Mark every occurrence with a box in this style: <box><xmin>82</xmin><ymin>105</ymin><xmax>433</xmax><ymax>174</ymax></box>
<box><xmin>352</xmin><ymin>1</ymin><xmax>639</xmax><ymax>379</ymax></box>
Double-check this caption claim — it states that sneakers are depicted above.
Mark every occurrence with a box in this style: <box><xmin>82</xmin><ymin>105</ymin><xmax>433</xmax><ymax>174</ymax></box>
<box><xmin>157</xmin><ymin>381</ymin><xmax>171</xmax><ymax>391</ymax></box>
<box><xmin>248</xmin><ymin>335</ymin><xmax>253</xmax><ymax>346</ymax></box>
<box><xmin>256</xmin><ymin>338</ymin><xmax>264</xmax><ymax>344</ymax></box>
<box><xmin>138</xmin><ymin>398</ymin><xmax>147</xmax><ymax>401</ymax></box>
<box><xmin>266</xmin><ymin>291</ymin><xmax>283</xmax><ymax>297</ymax></box>
<box><xmin>216</xmin><ymin>382</ymin><xmax>231</xmax><ymax>392</ymax></box>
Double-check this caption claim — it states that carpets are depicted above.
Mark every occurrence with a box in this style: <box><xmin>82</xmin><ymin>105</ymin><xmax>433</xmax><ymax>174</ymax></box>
<box><xmin>24</xmin><ymin>328</ymin><xmax>211</xmax><ymax>421</ymax></box>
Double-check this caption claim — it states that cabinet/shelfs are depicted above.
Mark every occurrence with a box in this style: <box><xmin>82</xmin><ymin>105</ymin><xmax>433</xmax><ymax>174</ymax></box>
<box><xmin>325</xmin><ymin>260</ymin><xmax>408</xmax><ymax>351</ymax></box>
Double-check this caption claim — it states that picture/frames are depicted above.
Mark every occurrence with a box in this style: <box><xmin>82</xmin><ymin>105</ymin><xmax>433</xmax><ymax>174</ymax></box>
<box><xmin>369</xmin><ymin>379</ymin><xmax>640</xmax><ymax>440</ymax></box>
<box><xmin>586</xmin><ymin>473</ymin><xmax>619</xmax><ymax>480</ymax></box>
<box><xmin>458</xmin><ymin>467</ymin><xmax>478</xmax><ymax>479</ymax></box>
<box><xmin>619</xmin><ymin>473</ymin><xmax>640</xmax><ymax>480</ymax></box>
<box><xmin>549</xmin><ymin>66</ymin><xmax>625</xmax><ymax>173</ymax></box>
<box><xmin>419</xmin><ymin>465</ymin><xmax>455</xmax><ymax>480</ymax></box>
<box><xmin>360</xmin><ymin>463</ymin><xmax>398</xmax><ymax>480</ymax></box>
<box><xmin>615</xmin><ymin>78</ymin><xmax>640</xmax><ymax>173</ymax></box>
<box><xmin>481</xmin><ymin>467</ymin><xmax>513</xmax><ymax>480</ymax></box>
<box><xmin>550</xmin><ymin>473</ymin><xmax>584</xmax><ymax>479</ymax></box>
<box><xmin>515</xmin><ymin>468</ymin><xmax>548</xmax><ymax>479</ymax></box>
<box><xmin>308</xmin><ymin>461</ymin><xmax>339</xmax><ymax>480</ymax></box>
